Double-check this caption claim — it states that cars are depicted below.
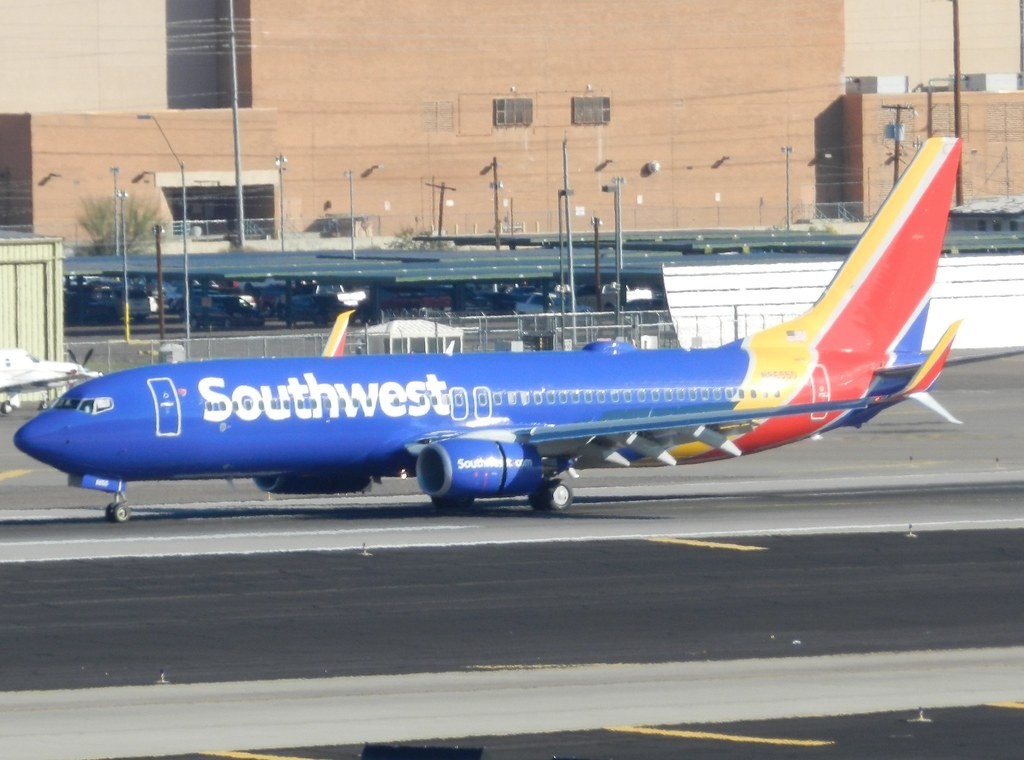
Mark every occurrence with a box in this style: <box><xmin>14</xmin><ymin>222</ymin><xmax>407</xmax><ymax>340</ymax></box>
<box><xmin>64</xmin><ymin>275</ymin><xmax>667</xmax><ymax>331</ymax></box>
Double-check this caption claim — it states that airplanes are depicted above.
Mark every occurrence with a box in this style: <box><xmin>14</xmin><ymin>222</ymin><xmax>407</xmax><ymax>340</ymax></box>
<box><xmin>0</xmin><ymin>346</ymin><xmax>105</xmax><ymax>415</ymax></box>
<box><xmin>13</xmin><ymin>135</ymin><xmax>967</xmax><ymax>523</ymax></box>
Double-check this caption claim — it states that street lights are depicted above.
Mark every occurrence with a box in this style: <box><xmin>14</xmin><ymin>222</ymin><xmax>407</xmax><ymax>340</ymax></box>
<box><xmin>273</xmin><ymin>152</ymin><xmax>287</xmax><ymax>252</ymax></box>
<box><xmin>557</xmin><ymin>190</ymin><xmax>576</xmax><ymax>343</ymax></box>
<box><xmin>131</xmin><ymin>170</ymin><xmax>166</xmax><ymax>365</ymax></box>
<box><xmin>479</xmin><ymin>156</ymin><xmax>500</xmax><ymax>252</ymax></box>
<box><xmin>781</xmin><ymin>146</ymin><xmax>793</xmax><ymax>232</ymax></box>
<box><xmin>601</xmin><ymin>177</ymin><xmax>625</xmax><ymax>336</ymax></box>
<box><xmin>137</xmin><ymin>113</ymin><xmax>190</xmax><ymax>360</ymax></box>
<box><xmin>345</xmin><ymin>169</ymin><xmax>356</xmax><ymax>260</ymax></box>
